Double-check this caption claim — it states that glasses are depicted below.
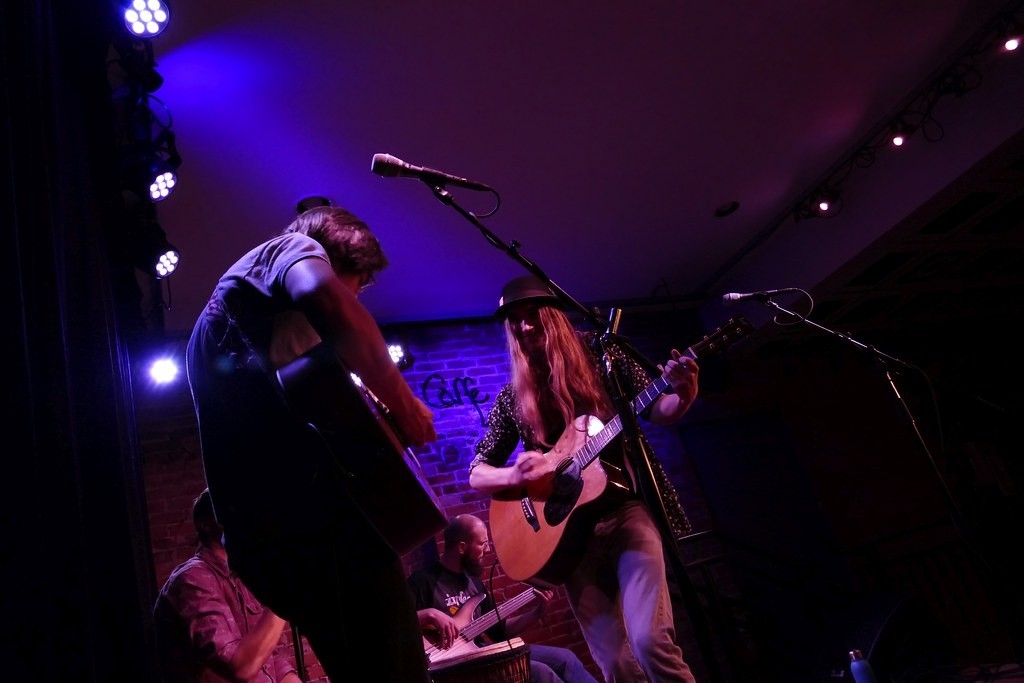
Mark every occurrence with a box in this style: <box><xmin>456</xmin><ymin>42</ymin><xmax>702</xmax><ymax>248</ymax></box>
<box><xmin>357</xmin><ymin>273</ymin><xmax>377</xmax><ymax>297</ymax></box>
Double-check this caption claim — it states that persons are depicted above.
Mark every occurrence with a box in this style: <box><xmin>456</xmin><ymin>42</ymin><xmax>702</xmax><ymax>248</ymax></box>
<box><xmin>469</xmin><ymin>272</ymin><xmax>700</xmax><ymax>683</ymax></box>
<box><xmin>416</xmin><ymin>512</ymin><xmax>600</xmax><ymax>682</ymax></box>
<box><xmin>149</xmin><ymin>195</ymin><xmax>461</xmax><ymax>682</ymax></box>
<box><xmin>151</xmin><ymin>489</ymin><xmax>300</xmax><ymax>682</ymax></box>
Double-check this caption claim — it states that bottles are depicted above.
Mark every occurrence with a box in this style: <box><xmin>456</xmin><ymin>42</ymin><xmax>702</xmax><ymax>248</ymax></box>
<box><xmin>849</xmin><ymin>650</ymin><xmax>874</xmax><ymax>683</ymax></box>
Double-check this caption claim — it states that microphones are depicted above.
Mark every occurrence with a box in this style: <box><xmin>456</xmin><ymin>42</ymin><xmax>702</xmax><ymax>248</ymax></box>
<box><xmin>371</xmin><ymin>152</ymin><xmax>484</xmax><ymax>193</ymax></box>
<box><xmin>723</xmin><ymin>289</ymin><xmax>778</xmax><ymax>304</ymax></box>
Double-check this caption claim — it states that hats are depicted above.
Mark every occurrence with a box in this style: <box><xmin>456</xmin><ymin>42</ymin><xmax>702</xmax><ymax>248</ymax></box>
<box><xmin>492</xmin><ymin>276</ymin><xmax>566</xmax><ymax>317</ymax></box>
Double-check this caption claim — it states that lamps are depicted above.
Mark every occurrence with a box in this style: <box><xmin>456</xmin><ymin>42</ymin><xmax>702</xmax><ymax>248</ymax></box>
<box><xmin>107</xmin><ymin>0</ymin><xmax>174</xmax><ymax>44</ymax></box>
<box><xmin>127</xmin><ymin>145</ymin><xmax>178</xmax><ymax>203</ymax></box>
<box><xmin>137</xmin><ymin>347</ymin><xmax>185</xmax><ymax>394</ymax></box>
<box><xmin>1001</xmin><ymin>33</ymin><xmax>1024</xmax><ymax>54</ymax></box>
<box><xmin>128</xmin><ymin>224</ymin><xmax>181</xmax><ymax>280</ymax></box>
<box><xmin>888</xmin><ymin>132</ymin><xmax>908</xmax><ymax>150</ymax></box>
<box><xmin>815</xmin><ymin>195</ymin><xmax>831</xmax><ymax>214</ymax></box>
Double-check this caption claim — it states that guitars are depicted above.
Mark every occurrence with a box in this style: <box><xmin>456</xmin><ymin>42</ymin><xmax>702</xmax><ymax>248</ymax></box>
<box><xmin>420</xmin><ymin>586</ymin><xmax>556</xmax><ymax>661</ymax></box>
<box><xmin>484</xmin><ymin>317</ymin><xmax>748</xmax><ymax>593</ymax></box>
<box><xmin>290</xmin><ymin>350</ymin><xmax>451</xmax><ymax>557</ymax></box>
